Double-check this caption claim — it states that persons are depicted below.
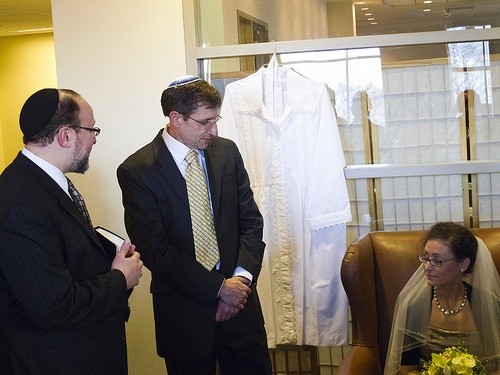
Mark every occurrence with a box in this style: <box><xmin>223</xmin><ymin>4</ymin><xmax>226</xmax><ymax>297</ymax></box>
<box><xmin>0</xmin><ymin>88</ymin><xmax>143</xmax><ymax>375</ymax></box>
<box><xmin>384</xmin><ymin>222</ymin><xmax>500</xmax><ymax>375</ymax></box>
<box><xmin>116</xmin><ymin>75</ymin><xmax>272</xmax><ymax>375</ymax></box>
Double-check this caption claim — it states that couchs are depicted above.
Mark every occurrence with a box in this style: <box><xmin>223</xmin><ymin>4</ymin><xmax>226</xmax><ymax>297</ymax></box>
<box><xmin>334</xmin><ymin>224</ymin><xmax>500</xmax><ymax>375</ymax></box>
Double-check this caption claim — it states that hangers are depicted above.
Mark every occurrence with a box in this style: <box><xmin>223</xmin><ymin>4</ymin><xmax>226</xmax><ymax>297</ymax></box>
<box><xmin>260</xmin><ymin>39</ymin><xmax>287</xmax><ymax>71</ymax></box>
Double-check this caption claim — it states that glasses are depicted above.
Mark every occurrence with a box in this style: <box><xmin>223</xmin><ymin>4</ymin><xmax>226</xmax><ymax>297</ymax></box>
<box><xmin>417</xmin><ymin>253</ymin><xmax>457</xmax><ymax>267</ymax></box>
<box><xmin>57</xmin><ymin>125</ymin><xmax>101</xmax><ymax>136</ymax></box>
<box><xmin>181</xmin><ymin>113</ymin><xmax>222</xmax><ymax>130</ymax></box>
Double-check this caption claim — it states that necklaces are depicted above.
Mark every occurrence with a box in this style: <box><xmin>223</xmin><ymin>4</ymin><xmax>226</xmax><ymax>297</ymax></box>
<box><xmin>434</xmin><ymin>286</ymin><xmax>467</xmax><ymax>316</ymax></box>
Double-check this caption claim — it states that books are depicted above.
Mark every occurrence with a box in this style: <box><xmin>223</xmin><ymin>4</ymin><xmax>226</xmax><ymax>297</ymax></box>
<box><xmin>94</xmin><ymin>226</ymin><xmax>125</xmax><ymax>262</ymax></box>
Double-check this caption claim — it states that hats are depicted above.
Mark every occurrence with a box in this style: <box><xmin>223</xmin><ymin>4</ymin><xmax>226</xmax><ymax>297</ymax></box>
<box><xmin>167</xmin><ymin>75</ymin><xmax>202</xmax><ymax>88</ymax></box>
<box><xmin>19</xmin><ymin>88</ymin><xmax>60</xmax><ymax>143</ymax></box>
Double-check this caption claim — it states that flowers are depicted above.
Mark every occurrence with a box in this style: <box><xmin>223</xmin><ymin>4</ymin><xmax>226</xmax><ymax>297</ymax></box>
<box><xmin>418</xmin><ymin>338</ymin><xmax>485</xmax><ymax>375</ymax></box>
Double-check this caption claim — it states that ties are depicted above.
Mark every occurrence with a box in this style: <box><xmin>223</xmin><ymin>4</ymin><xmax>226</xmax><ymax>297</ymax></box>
<box><xmin>64</xmin><ymin>175</ymin><xmax>95</xmax><ymax>233</ymax></box>
<box><xmin>183</xmin><ymin>149</ymin><xmax>220</xmax><ymax>273</ymax></box>
